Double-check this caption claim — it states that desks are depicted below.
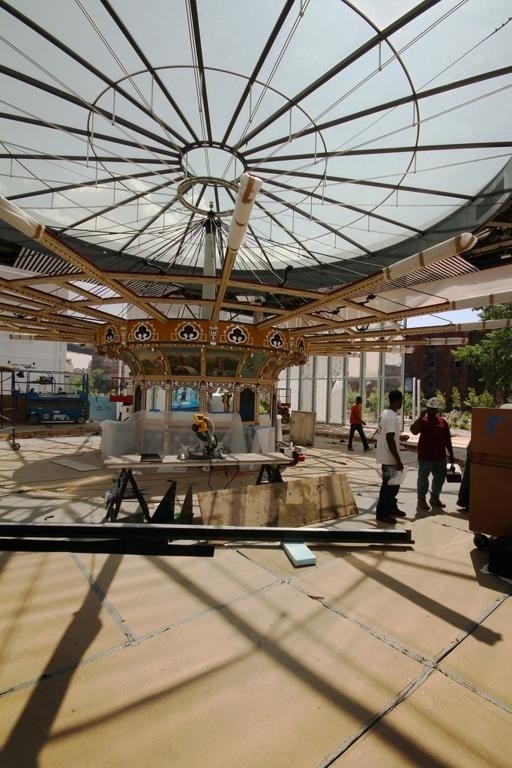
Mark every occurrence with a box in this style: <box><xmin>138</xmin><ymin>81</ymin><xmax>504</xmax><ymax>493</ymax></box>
<box><xmin>104</xmin><ymin>448</ymin><xmax>291</xmax><ymax>524</ymax></box>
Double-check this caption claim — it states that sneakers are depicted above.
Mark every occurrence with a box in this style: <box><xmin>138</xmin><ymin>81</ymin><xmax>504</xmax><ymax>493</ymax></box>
<box><xmin>418</xmin><ymin>500</ymin><xmax>448</xmax><ymax>510</ymax></box>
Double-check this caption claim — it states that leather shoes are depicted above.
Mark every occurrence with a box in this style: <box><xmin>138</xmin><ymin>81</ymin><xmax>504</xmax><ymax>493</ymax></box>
<box><xmin>379</xmin><ymin>508</ymin><xmax>406</xmax><ymax>523</ymax></box>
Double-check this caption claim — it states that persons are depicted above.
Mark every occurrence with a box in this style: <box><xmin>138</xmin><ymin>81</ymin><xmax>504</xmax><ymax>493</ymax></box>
<box><xmin>348</xmin><ymin>397</ymin><xmax>372</xmax><ymax>452</ymax></box>
<box><xmin>410</xmin><ymin>398</ymin><xmax>454</xmax><ymax>510</ymax></box>
<box><xmin>376</xmin><ymin>390</ymin><xmax>406</xmax><ymax>524</ymax></box>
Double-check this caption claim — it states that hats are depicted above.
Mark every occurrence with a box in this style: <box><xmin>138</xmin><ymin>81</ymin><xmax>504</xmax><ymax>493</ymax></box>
<box><xmin>426</xmin><ymin>398</ymin><xmax>444</xmax><ymax>409</ymax></box>
